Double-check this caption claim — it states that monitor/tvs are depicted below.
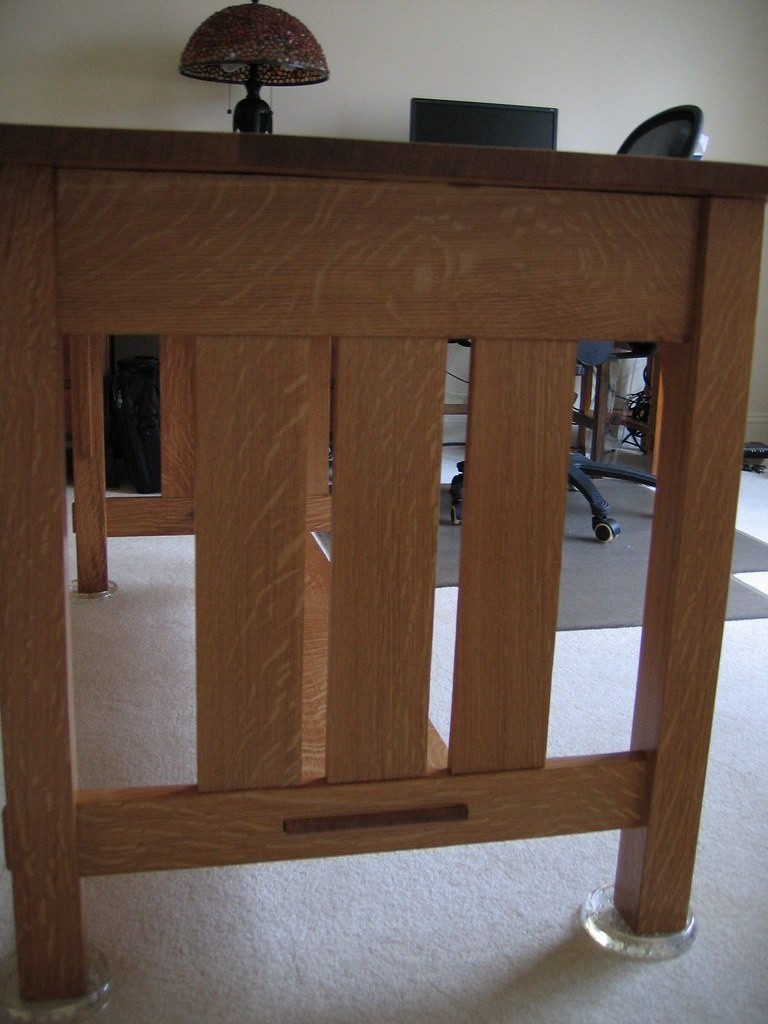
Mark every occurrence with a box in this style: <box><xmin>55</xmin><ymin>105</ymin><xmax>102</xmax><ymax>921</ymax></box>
<box><xmin>410</xmin><ymin>98</ymin><xmax>559</xmax><ymax>152</ymax></box>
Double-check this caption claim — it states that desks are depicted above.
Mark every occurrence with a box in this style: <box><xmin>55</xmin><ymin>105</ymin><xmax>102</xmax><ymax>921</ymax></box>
<box><xmin>0</xmin><ymin>123</ymin><xmax>768</xmax><ymax>1024</ymax></box>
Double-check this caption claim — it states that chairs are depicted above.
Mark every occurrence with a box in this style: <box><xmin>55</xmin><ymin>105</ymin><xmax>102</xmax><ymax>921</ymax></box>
<box><xmin>447</xmin><ymin>102</ymin><xmax>705</xmax><ymax>542</ymax></box>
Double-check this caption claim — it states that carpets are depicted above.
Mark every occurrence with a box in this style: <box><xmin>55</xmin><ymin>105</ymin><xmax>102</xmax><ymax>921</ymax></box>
<box><xmin>431</xmin><ymin>480</ymin><xmax>768</xmax><ymax>632</ymax></box>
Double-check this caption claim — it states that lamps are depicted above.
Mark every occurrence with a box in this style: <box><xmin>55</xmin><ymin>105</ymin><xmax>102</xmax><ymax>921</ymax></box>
<box><xmin>177</xmin><ymin>1</ymin><xmax>330</xmax><ymax>134</ymax></box>
